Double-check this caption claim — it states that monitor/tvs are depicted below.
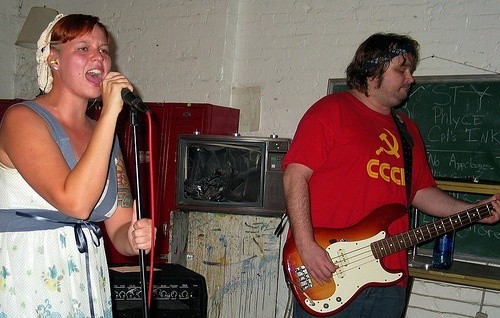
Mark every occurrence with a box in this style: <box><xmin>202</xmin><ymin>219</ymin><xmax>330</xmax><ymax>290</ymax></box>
<box><xmin>176</xmin><ymin>134</ymin><xmax>293</xmax><ymax>218</ymax></box>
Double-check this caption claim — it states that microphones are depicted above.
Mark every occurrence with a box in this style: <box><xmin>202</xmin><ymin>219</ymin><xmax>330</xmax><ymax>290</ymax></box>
<box><xmin>121</xmin><ymin>89</ymin><xmax>150</xmax><ymax>114</ymax></box>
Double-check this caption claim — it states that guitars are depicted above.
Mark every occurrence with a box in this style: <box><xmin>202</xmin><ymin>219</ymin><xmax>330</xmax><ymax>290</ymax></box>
<box><xmin>281</xmin><ymin>200</ymin><xmax>500</xmax><ymax>318</ymax></box>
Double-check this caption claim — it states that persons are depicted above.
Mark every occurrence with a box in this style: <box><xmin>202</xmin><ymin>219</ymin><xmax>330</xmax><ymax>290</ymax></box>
<box><xmin>282</xmin><ymin>33</ymin><xmax>500</xmax><ymax>318</ymax></box>
<box><xmin>0</xmin><ymin>14</ymin><xmax>158</xmax><ymax>317</ymax></box>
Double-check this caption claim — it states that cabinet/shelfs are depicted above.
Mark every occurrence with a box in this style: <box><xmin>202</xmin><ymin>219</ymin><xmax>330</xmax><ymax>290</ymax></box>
<box><xmin>89</xmin><ymin>103</ymin><xmax>240</xmax><ymax>268</ymax></box>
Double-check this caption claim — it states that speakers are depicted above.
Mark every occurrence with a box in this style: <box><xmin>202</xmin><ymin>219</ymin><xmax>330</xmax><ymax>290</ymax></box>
<box><xmin>109</xmin><ymin>262</ymin><xmax>208</xmax><ymax>318</ymax></box>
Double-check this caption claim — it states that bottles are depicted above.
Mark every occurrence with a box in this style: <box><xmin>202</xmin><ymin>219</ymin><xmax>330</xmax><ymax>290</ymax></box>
<box><xmin>432</xmin><ymin>230</ymin><xmax>455</xmax><ymax>272</ymax></box>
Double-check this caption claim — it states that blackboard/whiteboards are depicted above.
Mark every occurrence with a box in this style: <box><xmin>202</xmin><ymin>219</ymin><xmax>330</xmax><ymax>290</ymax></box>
<box><xmin>329</xmin><ymin>74</ymin><xmax>500</xmax><ymax>267</ymax></box>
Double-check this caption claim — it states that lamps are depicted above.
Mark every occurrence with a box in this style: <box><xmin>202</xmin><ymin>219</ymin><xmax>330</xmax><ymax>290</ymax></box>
<box><xmin>15</xmin><ymin>6</ymin><xmax>59</xmax><ymax>97</ymax></box>
